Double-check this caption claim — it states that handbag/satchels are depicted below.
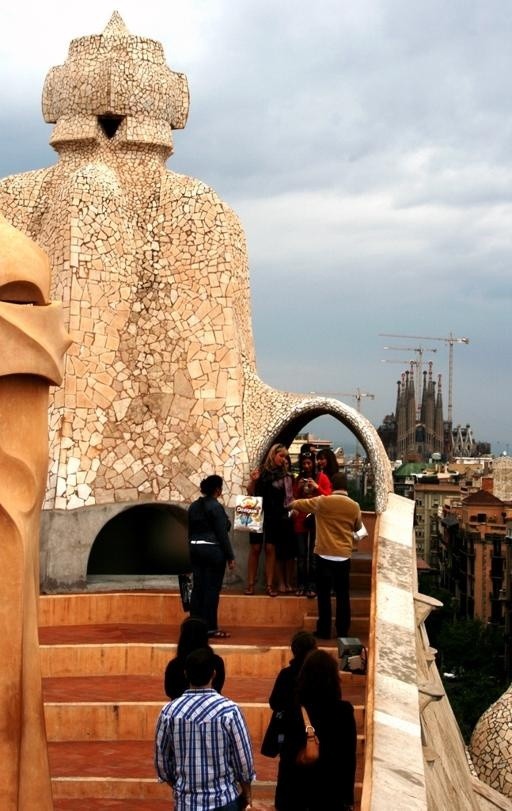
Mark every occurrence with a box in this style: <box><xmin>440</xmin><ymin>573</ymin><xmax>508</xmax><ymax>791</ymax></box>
<box><xmin>298</xmin><ymin>735</ymin><xmax>320</xmax><ymax>765</ymax></box>
<box><xmin>177</xmin><ymin>574</ymin><xmax>194</xmax><ymax>612</ymax></box>
<box><xmin>234</xmin><ymin>494</ymin><xmax>264</xmax><ymax>533</ymax></box>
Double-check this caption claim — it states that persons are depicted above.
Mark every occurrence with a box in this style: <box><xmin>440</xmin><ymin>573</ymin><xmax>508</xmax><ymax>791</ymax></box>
<box><xmin>242</xmin><ymin>442</ymin><xmax>339</xmax><ymax>598</ymax></box>
<box><xmin>163</xmin><ymin>615</ymin><xmax>226</xmax><ymax>701</ymax></box>
<box><xmin>188</xmin><ymin>474</ymin><xmax>235</xmax><ymax>638</ymax></box>
<box><xmin>153</xmin><ymin>650</ymin><xmax>257</xmax><ymax>811</ymax></box>
<box><xmin>283</xmin><ymin>472</ymin><xmax>369</xmax><ymax>640</ymax></box>
<box><xmin>275</xmin><ymin>650</ymin><xmax>357</xmax><ymax>811</ymax></box>
<box><xmin>269</xmin><ymin>629</ymin><xmax>319</xmax><ymax>714</ymax></box>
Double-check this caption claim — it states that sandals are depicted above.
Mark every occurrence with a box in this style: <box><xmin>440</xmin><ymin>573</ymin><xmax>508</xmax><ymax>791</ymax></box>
<box><xmin>246</xmin><ymin>586</ymin><xmax>316</xmax><ymax>598</ymax></box>
<box><xmin>209</xmin><ymin>630</ymin><xmax>229</xmax><ymax>638</ymax></box>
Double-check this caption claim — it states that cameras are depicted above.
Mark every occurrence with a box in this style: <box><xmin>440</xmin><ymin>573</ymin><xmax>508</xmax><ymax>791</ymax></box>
<box><xmin>303</xmin><ymin>478</ymin><xmax>309</xmax><ymax>486</ymax></box>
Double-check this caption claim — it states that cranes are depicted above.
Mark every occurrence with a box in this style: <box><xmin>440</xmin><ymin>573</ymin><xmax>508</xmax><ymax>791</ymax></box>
<box><xmin>379</xmin><ymin>332</ymin><xmax>469</xmax><ymax>421</ymax></box>
<box><xmin>309</xmin><ymin>388</ymin><xmax>375</xmax><ymax>459</ymax></box>
